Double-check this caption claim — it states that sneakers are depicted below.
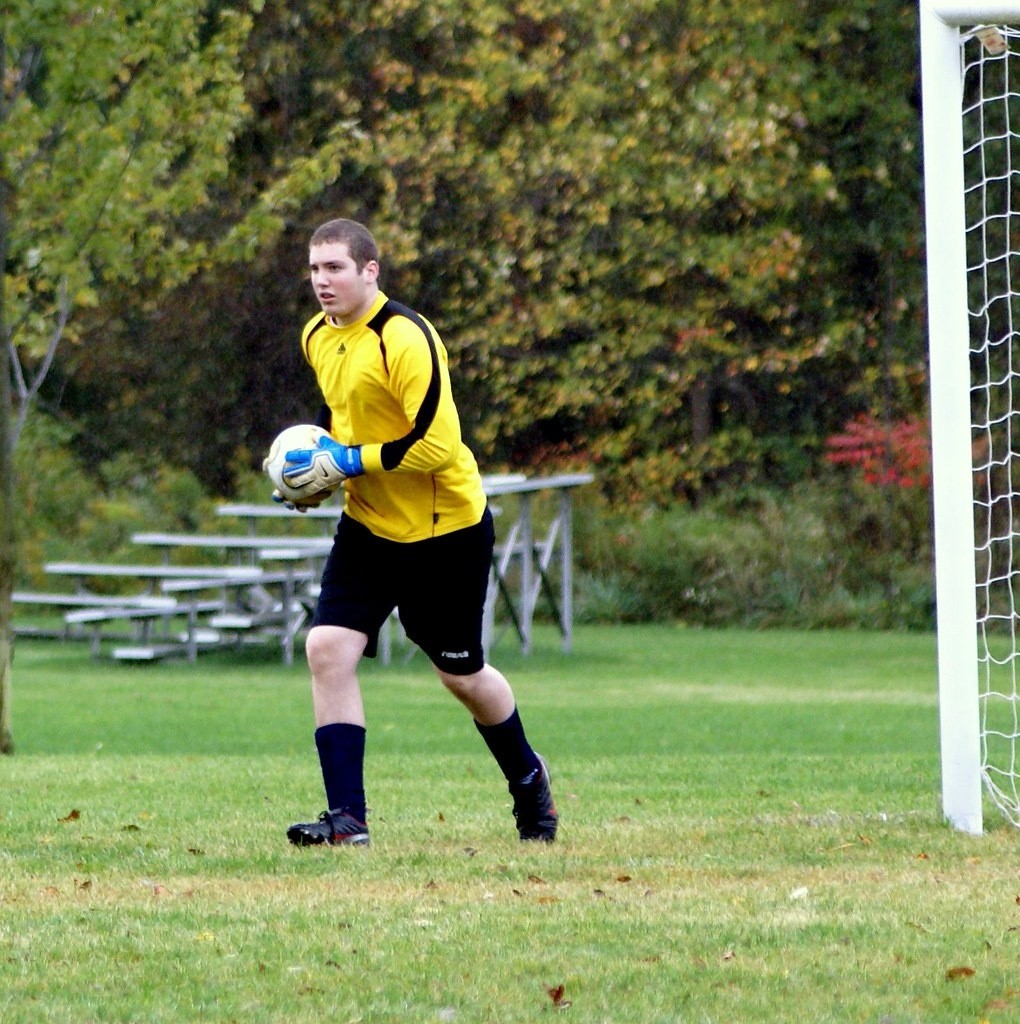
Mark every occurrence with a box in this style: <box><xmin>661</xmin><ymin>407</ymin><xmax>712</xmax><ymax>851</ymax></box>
<box><xmin>286</xmin><ymin>808</ymin><xmax>370</xmax><ymax>847</ymax></box>
<box><xmin>508</xmin><ymin>752</ymin><xmax>557</xmax><ymax>847</ymax></box>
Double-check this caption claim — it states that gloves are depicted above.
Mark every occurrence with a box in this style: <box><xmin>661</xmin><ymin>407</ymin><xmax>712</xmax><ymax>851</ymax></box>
<box><xmin>262</xmin><ymin>458</ymin><xmax>333</xmax><ymax>512</ymax></box>
<box><xmin>284</xmin><ymin>436</ymin><xmax>364</xmax><ymax>497</ymax></box>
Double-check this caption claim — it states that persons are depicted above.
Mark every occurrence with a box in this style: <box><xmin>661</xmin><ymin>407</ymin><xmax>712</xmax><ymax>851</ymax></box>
<box><xmin>272</xmin><ymin>218</ymin><xmax>558</xmax><ymax>847</ymax></box>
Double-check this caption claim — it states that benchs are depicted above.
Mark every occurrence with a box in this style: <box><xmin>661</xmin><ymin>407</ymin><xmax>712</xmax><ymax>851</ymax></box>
<box><xmin>9</xmin><ymin>474</ymin><xmax>596</xmax><ymax>667</ymax></box>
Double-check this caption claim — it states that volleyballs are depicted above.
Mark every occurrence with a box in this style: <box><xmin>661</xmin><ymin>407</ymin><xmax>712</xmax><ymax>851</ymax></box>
<box><xmin>263</xmin><ymin>421</ymin><xmax>347</xmax><ymax>505</ymax></box>
